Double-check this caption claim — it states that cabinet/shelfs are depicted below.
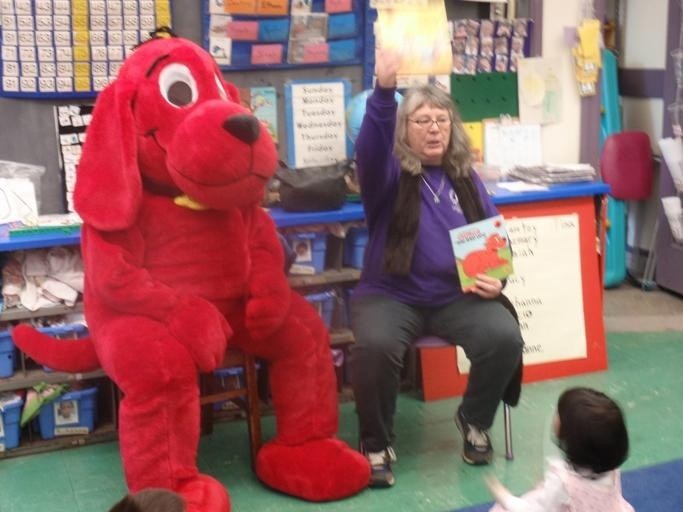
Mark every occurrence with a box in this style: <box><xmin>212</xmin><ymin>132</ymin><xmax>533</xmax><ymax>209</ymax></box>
<box><xmin>0</xmin><ymin>168</ymin><xmax>610</xmax><ymax>459</ymax></box>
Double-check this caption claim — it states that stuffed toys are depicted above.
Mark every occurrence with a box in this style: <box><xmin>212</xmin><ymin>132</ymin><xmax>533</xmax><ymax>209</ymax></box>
<box><xmin>12</xmin><ymin>36</ymin><xmax>371</xmax><ymax>512</ymax></box>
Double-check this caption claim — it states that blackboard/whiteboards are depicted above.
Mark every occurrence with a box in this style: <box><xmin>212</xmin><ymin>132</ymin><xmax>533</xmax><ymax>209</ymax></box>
<box><xmin>456</xmin><ymin>213</ymin><xmax>588</xmax><ymax>375</ymax></box>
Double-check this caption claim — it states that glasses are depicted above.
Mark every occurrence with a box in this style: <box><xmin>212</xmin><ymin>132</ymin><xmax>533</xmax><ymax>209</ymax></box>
<box><xmin>409</xmin><ymin>115</ymin><xmax>450</xmax><ymax>129</ymax></box>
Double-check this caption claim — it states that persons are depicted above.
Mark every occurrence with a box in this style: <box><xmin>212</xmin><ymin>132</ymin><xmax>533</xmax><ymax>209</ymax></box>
<box><xmin>349</xmin><ymin>39</ymin><xmax>524</xmax><ymax>489</ymax></box>
<box><xmin>57</xmin><ymin>401</ymin><xmax>77</xmax><ymax>424</ymax></box>
<box><xmin>108</xmin><ymin>490</ymin><xmax>184</xmax><ymax>512</ymax></box>
<box><xmin>484</xmin><ymin>388</ymin><xmax>636</xmax><ymax>512</ymax></box>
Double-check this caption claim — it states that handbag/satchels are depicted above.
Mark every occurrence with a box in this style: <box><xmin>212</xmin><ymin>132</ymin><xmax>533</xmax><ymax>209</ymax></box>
<box><xmin>274</xmin><ymin>160</ymin><xmax>351</xmax><ymax>212</ymax></box>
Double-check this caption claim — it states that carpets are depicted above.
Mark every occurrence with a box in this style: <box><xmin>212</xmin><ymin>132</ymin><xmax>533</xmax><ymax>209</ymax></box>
<box><xmin>450</xmin><ymin>458</ymin><xmax>683</xmax><ymax>512</ymax></box>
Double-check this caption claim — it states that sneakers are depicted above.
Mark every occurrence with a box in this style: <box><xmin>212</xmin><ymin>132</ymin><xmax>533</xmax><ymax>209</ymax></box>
<box><xmin>358</xmin><ymin>440</ymin><xmax>396</xmax><ymax>487</ymax></box>
<box><xmin>455</xmin><ymin>404</ymin><xmax>492</xmax><ymax>466</ymax></box>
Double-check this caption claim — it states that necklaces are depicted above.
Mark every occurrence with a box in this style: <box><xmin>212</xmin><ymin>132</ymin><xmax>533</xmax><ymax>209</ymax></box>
<box><xmin>420</xmin><ymin>171</ymin><xmax>447</xmax><ymax>204</ymax></box>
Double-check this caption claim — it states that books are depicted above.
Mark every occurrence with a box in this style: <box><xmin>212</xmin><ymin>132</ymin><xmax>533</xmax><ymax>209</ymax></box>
<box><xmin>449</xmin><ymin>214</ymin><xmax>515</xmax><ymax>294</ymax></box>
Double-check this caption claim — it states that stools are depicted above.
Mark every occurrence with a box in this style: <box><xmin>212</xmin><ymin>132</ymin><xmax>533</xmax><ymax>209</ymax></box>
<box><xmin>111</xmin><ymin>347</ymin><xmax>261</xmax><ymax>477</ymax></box>
<box><xmin>342</xmin><ymin>283</ymin><xmax>513</xmax><ymax>463</ymax></box>
